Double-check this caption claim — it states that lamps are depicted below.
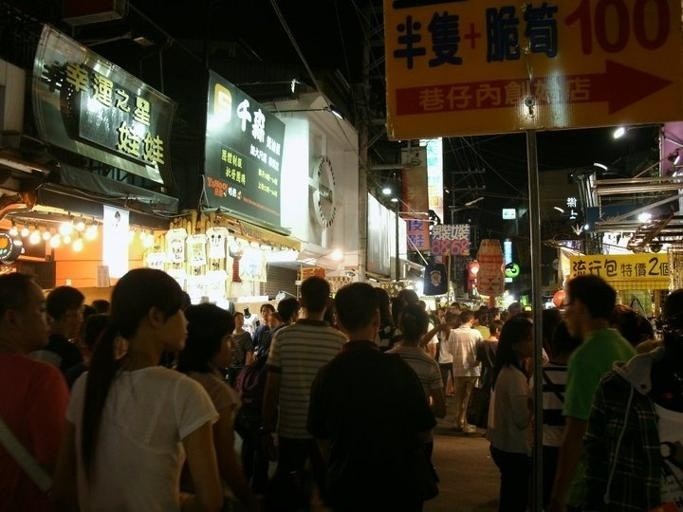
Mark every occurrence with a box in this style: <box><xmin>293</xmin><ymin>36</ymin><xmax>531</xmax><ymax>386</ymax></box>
<box><xmin>269</xmin><ymin>103</ymin><xmax>345</xmax><ymax>122</ymax></box>
<box><xmin>666</xmin><ymin>153</ymin><xmax>680</xmax><ymax>177</ymax></box>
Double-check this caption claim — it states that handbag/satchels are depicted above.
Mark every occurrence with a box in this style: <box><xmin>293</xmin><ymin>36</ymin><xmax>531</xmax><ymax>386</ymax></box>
<box><xmin>466</xmin><ymin>385</ymin><xmax>489</xmax><ymax>427</ymax></box>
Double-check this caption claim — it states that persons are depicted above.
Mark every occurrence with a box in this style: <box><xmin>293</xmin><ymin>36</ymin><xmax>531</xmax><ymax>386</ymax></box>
<box><xmin>435</xmin><ymin>275</ymin><xmax>683</xmax><ymax>512</ymax></box>
<box><xmin>1</xmin><ymin>269</ymin><xmax>452</xmax><ymax>512</ymax></box>
